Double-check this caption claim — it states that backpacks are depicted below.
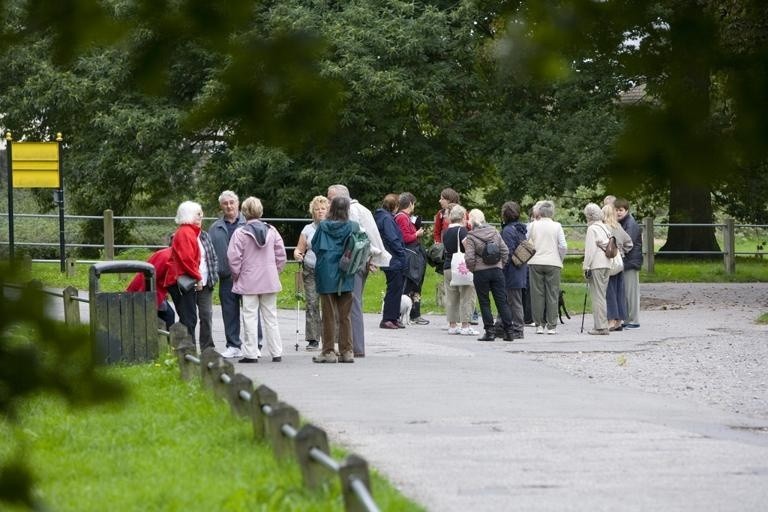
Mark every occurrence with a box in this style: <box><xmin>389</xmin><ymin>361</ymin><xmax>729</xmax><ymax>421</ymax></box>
<box><xmin>475</xmin><ymin>238</ymin><xmax>501</xmax><ymax>265</ymax></box>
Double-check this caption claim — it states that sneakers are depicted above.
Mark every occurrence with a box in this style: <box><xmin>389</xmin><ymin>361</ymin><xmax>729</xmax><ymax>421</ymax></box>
<box><xmin>588</xmin><ymin>324</ymin><xmax>640</xmax><ymax>335</ymax></box>
<box><xmin>380</xmin><ymin>317</ymin><xmax>556</xmax><ymax>340</ymax></box>
<box><xmin>222</xmin><ymin>341</ymin><xmax>364</xmax><ymax>363</ymax></box>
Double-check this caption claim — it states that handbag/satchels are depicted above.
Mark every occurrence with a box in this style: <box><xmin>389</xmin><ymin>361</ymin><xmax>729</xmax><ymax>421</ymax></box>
<box><xmin>512</xmin><ymin>240</ymin><xmax>536</xmax><ymax>267</ymax></box>
<box><xmin>450</xmin><ymin>253</ymin><xmax>474</xmax><ymax>286</ymax></box>
<box><xmin>339</xmin><ymin>230</ymin><xmax>370</xmax><ymax>276</ymax></box>
<box><xmin>596</xmin><ymin>224</ymin><xmax>617</xmax><ymax>257</ymax></box>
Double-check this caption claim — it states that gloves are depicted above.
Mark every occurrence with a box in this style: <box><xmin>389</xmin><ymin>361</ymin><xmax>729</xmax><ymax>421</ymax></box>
<box><xmin>586</xmin><ymin>270</ymin><xmax>593</xmax><ymax>279</ymax></box>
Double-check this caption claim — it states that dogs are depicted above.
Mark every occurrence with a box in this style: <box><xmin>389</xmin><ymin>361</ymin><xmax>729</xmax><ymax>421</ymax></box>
<box><xmin>381</xmin><ymin>294</ymin><xmax>413</xmax><ymax>326</ymax></box>
<box><xmin>543</xmin><ymin>290</ymin><xmax>572</xmax><ymax>325</ymax></box>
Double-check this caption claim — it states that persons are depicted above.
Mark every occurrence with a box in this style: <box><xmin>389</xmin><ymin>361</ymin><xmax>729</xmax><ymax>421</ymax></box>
<box><xmin>123</xmin><ymin>232</ymin><xmax>177</xmax><ymax>344</ymax></box>
<box><xmin>583</xmin><ymin>195</ymin><xmax>642</xmax><ymax>335</ymax></box>
<box><xmin>312</xmin><ymin>196</ymin><xmax>361</xmax><ymax>364</ymax></box>
<box><xmin>393</xmin><ymin>192</ymin><xmax>430</xmax><ymax>326</ymax></box>
<box><xmin>208</xmin><ymin>189</ymin><xmax>265</xmax><ymax>359</ymax></box>
<box><xmin>327</xmin><ymin>184</ymin><xmax>392</xmax><ymax>358</ymax></box>
<box><xmin>196</xmin><ymin>228</ymin><xmax>219</xmax><ymax>353</ymax></box>
<box><xmin>432</xmin><ymin>188</ymin><xmax>567</xmax><ymax>342</ymax></box>
<box><xmin>292</xmin><ymin>194</ymin><xmax>331</xmax><ymax>352</ymax></box>
<box><xmin>226</xmin><ymin>196</ymin><xmax>287</xmax><ymax>364</ymax></box>
<box><xmin>373</xmin><ymin>194</ymin><xmax>408</xmax><ymax>330</ymax></box>
<box><xmin>172</xmin><ymin>202</ymin><xmax>203</xmax><ymax>352</ymax></box>
